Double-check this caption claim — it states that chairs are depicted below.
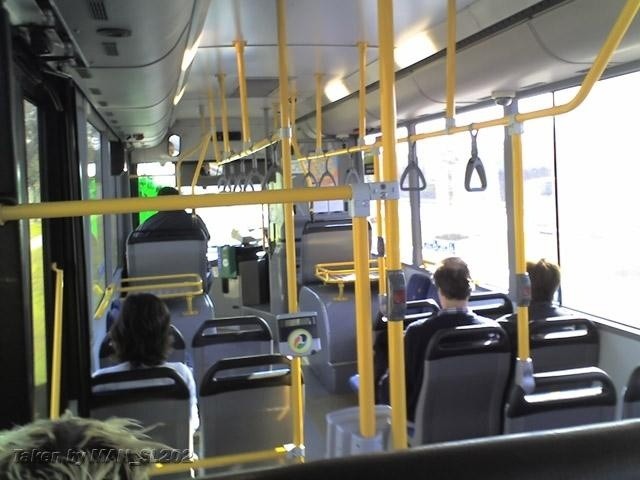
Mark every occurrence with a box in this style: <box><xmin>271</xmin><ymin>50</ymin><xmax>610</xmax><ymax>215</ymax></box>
<box><xmin>79</xmin><ymin>210</ymin><xmax>639</xmax><ymax>480</ymax></box>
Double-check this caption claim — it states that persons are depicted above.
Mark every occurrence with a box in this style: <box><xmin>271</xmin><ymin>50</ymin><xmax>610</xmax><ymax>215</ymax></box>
<box><xmin>91</xmin><ymin>293</ymin><xmax>200</xmax><ymax>432</ymax></box>
<box><xmin>377</xmin><ymin>257</ymin><xmax>503</xmax><ymax>422</ymax></box>
<box><xmin>496</xmin><ymin>260</ymin><xmax>587</xmax><ymax>337</ymax></box>
<box><xmin>0</xmin><ymin>408</ymin><xmax>173</xmax><ymax>480</ymax></box>
<box><xmin>168</xmin><ymin>142</ymin><xmax>175</xmax><ymax>155</ymax></box>
<box><xmin>201</xmin><ymin>163</ymin><xmax>210</xmax><ymax>176</ymax></box>
<box><xmin>133</xmin><ymin>187</ymin><xmax>210</xmax><ymax>273</ymax></box>
